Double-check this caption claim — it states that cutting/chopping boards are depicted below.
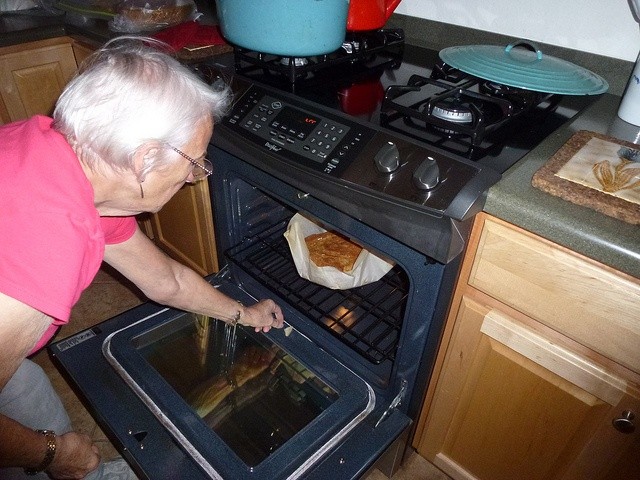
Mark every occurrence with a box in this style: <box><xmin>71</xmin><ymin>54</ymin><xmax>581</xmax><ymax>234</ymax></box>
<box><xmin>531</xmin><ymin>130</ymin><xmax>640</xmax><ymax>226</ymax></box>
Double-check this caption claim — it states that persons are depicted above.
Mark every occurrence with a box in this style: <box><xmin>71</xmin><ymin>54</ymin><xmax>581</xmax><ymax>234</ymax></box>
<box><xmin>0</xmin><ymin>37</ymin><xmax>285</xmax><ymax>476</ymax></box>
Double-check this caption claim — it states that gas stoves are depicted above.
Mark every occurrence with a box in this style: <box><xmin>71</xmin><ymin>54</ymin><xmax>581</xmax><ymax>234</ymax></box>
<box><xmin>202</xmin><ymin>29</ymin><xmax>601</xmax><ymax>221</ymax></box>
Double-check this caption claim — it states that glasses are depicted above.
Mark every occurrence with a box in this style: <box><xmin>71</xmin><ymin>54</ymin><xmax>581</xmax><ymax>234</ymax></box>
<box><xmin>163</xmin><ymin>140</ymin><xmax>213</xmax><ymax>181</ymax></box>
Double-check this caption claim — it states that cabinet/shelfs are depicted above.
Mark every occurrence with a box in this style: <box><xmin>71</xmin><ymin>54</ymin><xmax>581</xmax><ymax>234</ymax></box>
<box><xmin>408</xmin><ymin>117</ymin><xmax>639</xmax><ymax>479</ymax></box>
<box><xmin>150</xmin><ymin>155</ymin><xmax>219</xmax><ymax>279</ymax></box>
<box><xmin>1</xmin><ymin>36</ymin><xmax>78</xmax><ymax>125</ymax></box>
<box><xmin>72</xmin><ymin>40</ymin><xmax>153</xmax><ymax>239</ymax></box>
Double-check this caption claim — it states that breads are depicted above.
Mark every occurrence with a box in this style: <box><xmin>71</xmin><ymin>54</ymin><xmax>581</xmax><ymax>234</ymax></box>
<box><xmin>304</xmin><ymin>231</ymin><xmax>363</xmax><ymax>272</ymax></box>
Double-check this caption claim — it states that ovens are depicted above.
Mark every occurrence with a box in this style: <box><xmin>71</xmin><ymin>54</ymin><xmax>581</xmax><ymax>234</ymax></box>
<box><xmin>45</xmin><ymin>145</ymin><xmax>454</xmax><ymax>480</ymax></box>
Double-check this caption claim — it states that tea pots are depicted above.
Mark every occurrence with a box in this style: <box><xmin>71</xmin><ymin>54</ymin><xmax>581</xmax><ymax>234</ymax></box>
<box><xmin>347</xmin><ymin>1</ymin><xmax>402</xmax><ymax>30</ymax></box>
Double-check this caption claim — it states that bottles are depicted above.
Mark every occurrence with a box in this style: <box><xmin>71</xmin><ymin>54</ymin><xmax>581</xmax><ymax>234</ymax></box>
<box><xmin>606</xmin><ymin>51</ymin><xmax>640</xmax><ymax>128</ymax></box>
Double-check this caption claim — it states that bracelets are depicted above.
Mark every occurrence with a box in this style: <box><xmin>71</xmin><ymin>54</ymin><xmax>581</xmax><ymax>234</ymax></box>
<box><xmin>223</xmin><ymin>301</ymin><xmax>246</xmax><ymax>329</ymax></box>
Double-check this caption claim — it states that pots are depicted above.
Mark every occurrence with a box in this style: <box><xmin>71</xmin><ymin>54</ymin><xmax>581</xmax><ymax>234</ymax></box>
<box><xmin>217</xmin><ymin>1</ymin><xmax>350</xmax><ymax>55</ymax></box>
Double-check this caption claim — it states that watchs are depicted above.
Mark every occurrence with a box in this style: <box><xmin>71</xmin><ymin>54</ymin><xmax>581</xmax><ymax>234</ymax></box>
<box><xmin>24</xmin><ymin>427</ymin><xmax>56</xmax><ymax>476</ymax></box>
